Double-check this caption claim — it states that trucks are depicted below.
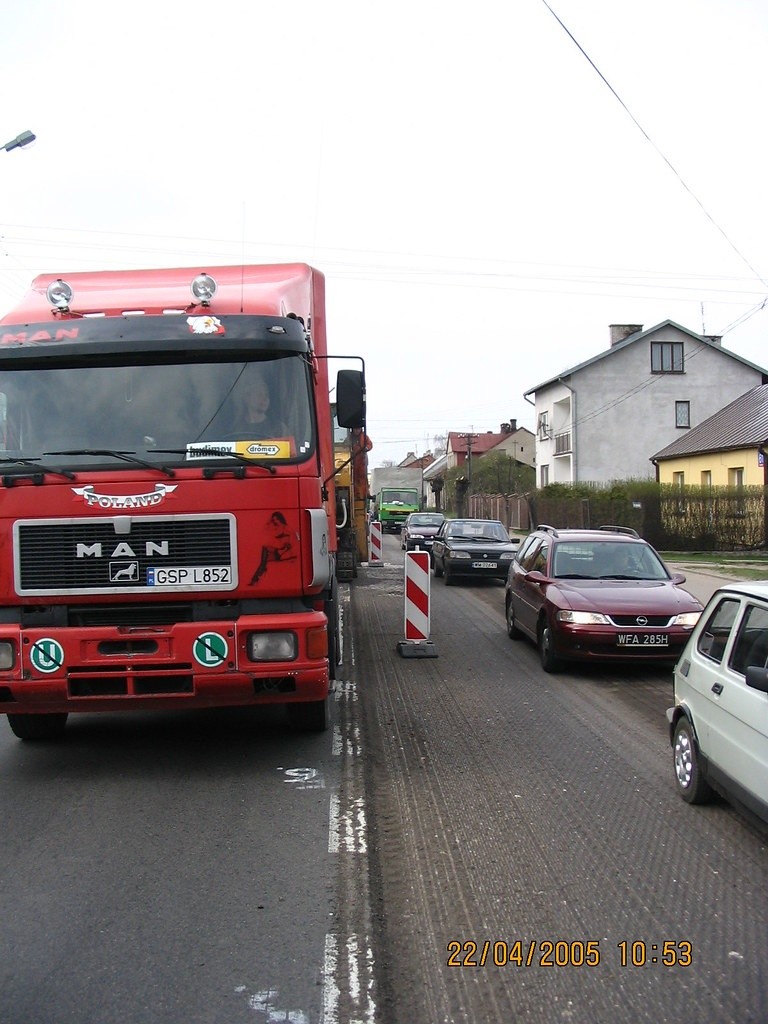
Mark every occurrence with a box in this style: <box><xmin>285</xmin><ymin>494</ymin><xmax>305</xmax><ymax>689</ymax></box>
<box><xmin>0</xmin><ymin>263</ymin><xmax>368</xmax><ymax>740</ymax></box>
<box><xmin>371</xmin><ymin>467</ymin><xmax>424</xmax><ymax>533</ymax></box>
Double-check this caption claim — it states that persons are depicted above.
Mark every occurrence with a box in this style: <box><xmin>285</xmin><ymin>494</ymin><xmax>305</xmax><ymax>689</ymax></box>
<box><xmin>603</xmin><ymin>545</ymin><xmax>643</xmax><ymax>576</ymax></box>
<box><xmin>482</xmin><ymin>524</ymin><xmax>498</xmax><ymax>539</ymax></box>
<box><xmin>384</xmin><ymin>493</ymin><xmax>415</xmax><ymax>502</ymax></box>
<box><xmin>209</xmin><ymin>376</ymin><xmax>291</xmax><ymax>442</ymax></box>
<box><xmin>248</xmin><ymin>511</ymin><xmax>295</xmax><ymax>585</ymax></box>
<box><xmin>344</xmin><ymin>427</ymin><xmax>372</xmax><ymax>501</ymax></box>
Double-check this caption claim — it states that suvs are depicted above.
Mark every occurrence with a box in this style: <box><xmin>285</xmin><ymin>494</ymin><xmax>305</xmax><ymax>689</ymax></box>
<box><xmin>504</xmin><ymin>523</ymin><xmax>707</xmax><ymax>675</ymax></box>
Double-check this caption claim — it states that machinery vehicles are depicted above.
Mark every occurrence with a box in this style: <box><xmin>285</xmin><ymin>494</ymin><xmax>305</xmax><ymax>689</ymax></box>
<box><xmin>328</xmin><ymin>397</ymin><xmax>372</xmax><ymax>582</ymax></box>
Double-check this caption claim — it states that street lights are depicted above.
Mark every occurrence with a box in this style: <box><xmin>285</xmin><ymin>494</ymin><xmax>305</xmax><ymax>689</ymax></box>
<box><xmin>457</xmin><ymin>432</ymin><xmax>480</xmax><ymax>519</ymax></box>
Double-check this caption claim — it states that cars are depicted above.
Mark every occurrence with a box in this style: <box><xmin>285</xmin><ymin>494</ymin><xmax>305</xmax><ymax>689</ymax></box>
<box><xmin>431</xmin><ymin>518</ymin><xmax>520</xmax><ymax>586</ymax></box>
<box><xmin>666</xmin><ymin>580</ymin><xmax>768</xmax><ymax>837</ymax></box>
<box><xmin>399</xmin><ymin>512</ymin><xmax>446</xmax><ymax>553</ymax></box>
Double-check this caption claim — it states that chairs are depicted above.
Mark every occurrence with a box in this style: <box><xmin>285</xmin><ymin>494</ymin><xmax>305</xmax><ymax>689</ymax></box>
<box><xmin>556</xmin><ymin>552</ymin><xmax>576</xmax><ymax>574</ymax></box>
<box><xmin>451</xmin><ymin>527</ymin><xmax>464</xmax><ymax>536</ymax></box>
